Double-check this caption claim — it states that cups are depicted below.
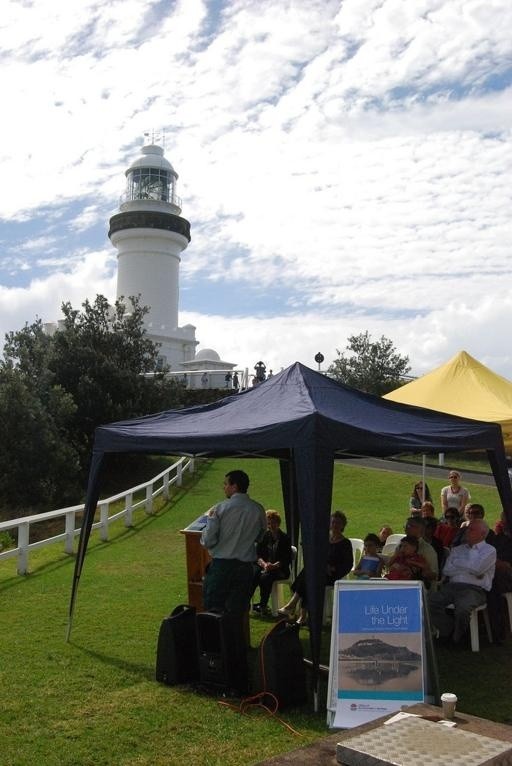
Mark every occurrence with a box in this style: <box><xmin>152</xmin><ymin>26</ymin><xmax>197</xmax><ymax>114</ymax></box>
<box><xmin>440</xmin><ymin>692</ymin><xmax>459</xmax><ymax>719</ymax></box>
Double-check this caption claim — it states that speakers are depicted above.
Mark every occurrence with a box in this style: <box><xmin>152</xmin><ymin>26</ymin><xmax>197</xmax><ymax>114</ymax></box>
<box><xmin>155</xmin><ymin>603</ymin><xmax>197</xmax><ymax>686</ymax></box>
<box><xmin>196</xmin><ymin>607</ymin><xmax>245</xmax><ymax>693</ymax></box>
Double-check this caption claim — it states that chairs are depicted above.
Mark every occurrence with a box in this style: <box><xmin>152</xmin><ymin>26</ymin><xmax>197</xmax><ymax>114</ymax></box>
<box><xmin>268</xmin><ymin>533</ymin><xmax>493</xmax><ymax>653</ymax></box>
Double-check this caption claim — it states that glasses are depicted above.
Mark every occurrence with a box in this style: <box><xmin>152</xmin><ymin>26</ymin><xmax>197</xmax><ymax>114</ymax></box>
<box><xmin>450</xmin><ymin>476</ymin><xmax>457</xmax><ymax>479</ymax></box>
<box><xmin>445</xmin><ymin>516</ymin><xmax>455</xmax><ymax>520</ymax></box>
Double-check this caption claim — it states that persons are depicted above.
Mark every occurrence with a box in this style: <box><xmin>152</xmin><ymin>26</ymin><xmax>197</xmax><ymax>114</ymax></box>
<box><xmin>441</xmin><ymin>470</ymin><xmax>469</xmax><ymax>521</ymax></box>
<box><xmin>200</xmin><ymin>372</ymin><xmax>209</xmax><ymax>389</ymax></box>
<box><xmin>179</xmin><ymin>373</ymin><xmax>188</xmax><ymax>390</ymax></box>
<box><xmin>223</xmin><ymin>371</ymin><xmax>232</xmax><ymax>388</ymax></box>
<box><xmin>271</xmin><ymin>510</ymin><xmax>354</xmax><ymax>624</ymax></box>
<box><xmin>352</xmin><ymin>501</ymin><xmax>512</xmax><ymax>645</ymax></box>
<box><xmin>251</xmin><ymin>360</ymin><xmax>274</xmax><ymax>387</ymax></box>
<box><xmin>232</xmin><ymin>372</ymin><xmax>239</xmax><ymax>389</ymax></box>
<box><xmin>409</xmin><ymin>480</ymin><xmax>433</xmax><ymax>518</ymax></box>
<box><xmin>252</xmin><ymin>509</ymin><xmax>292</xmax><ymax>613</ymax></box>
<box><xmin>198</xmin><ymin>469</ymin><xmax>267</xmax><ymax>615</ymax></box>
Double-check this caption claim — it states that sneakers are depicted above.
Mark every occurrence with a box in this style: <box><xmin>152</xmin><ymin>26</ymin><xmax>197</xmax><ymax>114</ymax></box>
<box><xmin>436</xmin><ymin>629</ymin><xmax>455</xmax><ymax>648</ymax></box>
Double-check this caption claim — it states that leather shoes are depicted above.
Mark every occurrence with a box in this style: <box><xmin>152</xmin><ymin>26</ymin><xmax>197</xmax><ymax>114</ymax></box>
<box><xmin>277</xmin><ymin>605</ymin><xmax>296</xmax><ymax>616</ymax></box>
<box><xmin>292</xmin><ymin>616</ymin><xmax>306</xmax><ymax>627</ymax></box>
